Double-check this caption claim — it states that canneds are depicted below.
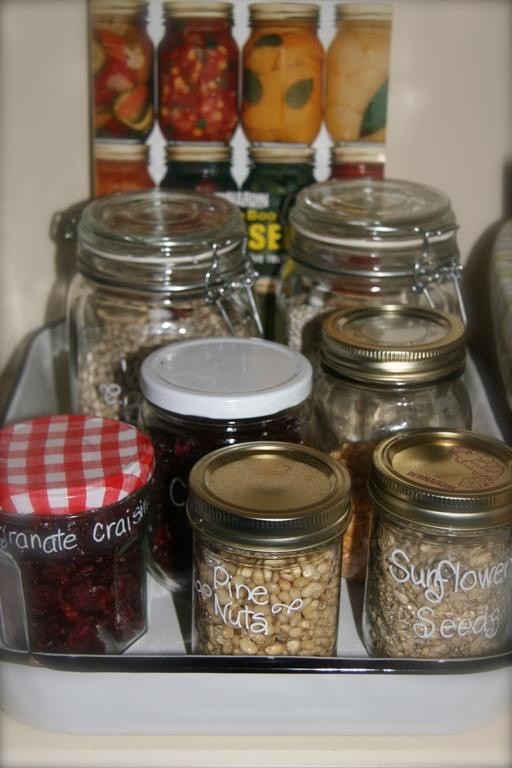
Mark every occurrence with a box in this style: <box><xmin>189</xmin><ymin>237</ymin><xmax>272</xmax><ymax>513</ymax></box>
<box><xmin>1</xmin><ymin>416</ymin><xmax>156</xmax><ymax>658</ymax></box>
<box><xmin>93</xmin><ymin>1</ymin><xmax>392</xmax><ymax>228</ymax></box>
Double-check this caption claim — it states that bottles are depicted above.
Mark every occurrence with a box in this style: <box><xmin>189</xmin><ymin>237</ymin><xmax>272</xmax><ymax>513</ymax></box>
<box><xmin>63</xmin><ymin>193</ymin><xmax>263</xmax><ymax>416</ymax></box>
<box><xmin>272</xmin><ymin>183</ymin><xmax>471</xmax><ymax>351</ymax></box>
